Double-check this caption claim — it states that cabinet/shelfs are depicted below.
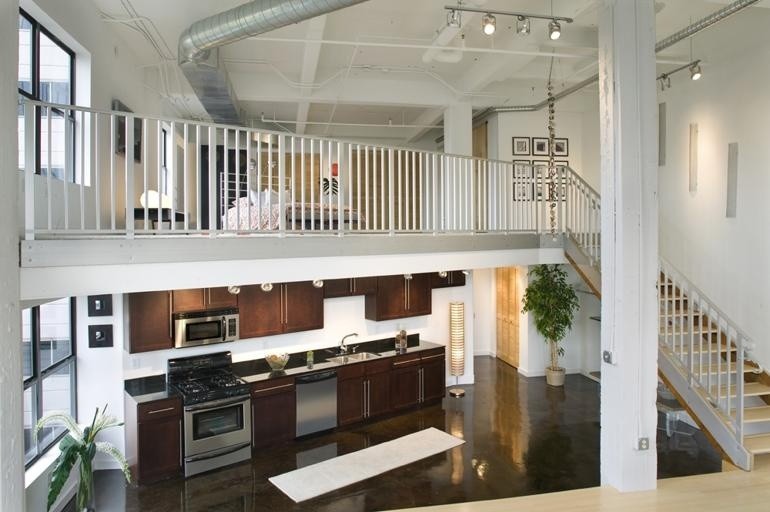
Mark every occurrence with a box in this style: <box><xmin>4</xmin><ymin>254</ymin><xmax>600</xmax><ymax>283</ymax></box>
<box><xmin>432</xmin><ymin>270</ymin><xmax>467</xmax><ymax>289</ymax></box>
<box><xmin>250</xmin><ymin>375</ymin><xmax>296</xmax><ymax>448</ymax></box>
<box><xmin>496</xmin><ymin>267</ymin><xmax>521</xmax><ymax>370</ymax></box>
<box><xmin>172</xmin><ymin>285</ymin><xmax>238</xmax><ymax>314</ymax></box>
<box><xmin>123</xmin><ymin>392</ymin><xmax>184</xmax><ymax>484</ymax></box>
<box><xmin>391</xmin><ymin>347</ymin><xmax>446</xmax><ymax>413</ymax></box>
<box><xmin>324</xmin><ymin>276</ymin><xmax>378</xmax><ymax>298</ymax></box>
<box><xmin>365</xmin><ymin>272</ymin><xmax>432</xmax><ymax>322</ymax></box>
<box><xmin>238</xmin><ymin>280</ymin><xmax>324</xmax><ymax>340</ymax></box>
<box><xmin>122</xmin><ymin>289</ymin><xmax>173</xmax><ymax>355</ymax></box>
<box><xmin>336</xmin><ymin>357</ymin><xmax>390</xmax><ymax>427</ymax></box>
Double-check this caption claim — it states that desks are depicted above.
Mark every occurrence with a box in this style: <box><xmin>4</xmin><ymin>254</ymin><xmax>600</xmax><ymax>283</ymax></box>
<box><xmin>127</xmin><ymin>209</ymin><xmax>191</xmax><ymax>236</ymax></box>
<box><xmin>589</xmin><ymin>371</ymin><xmax>686</xmax><ymax>437</ymax></box>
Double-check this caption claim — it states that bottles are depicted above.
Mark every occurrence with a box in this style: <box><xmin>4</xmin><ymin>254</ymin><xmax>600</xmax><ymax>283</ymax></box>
<box><xmin>399</xmin><ymin>330</ymin><xmax>407</xmax><ymax>351</ymax></box>
<box><xmin>395</xmin><ymin>335</ymin><xmax>400</xmax><ymax>350</ymax></box>
<box><xmin>307</xmin><ymin>349</ymin><xmax>314</xmax><ymax>369</ymax></box>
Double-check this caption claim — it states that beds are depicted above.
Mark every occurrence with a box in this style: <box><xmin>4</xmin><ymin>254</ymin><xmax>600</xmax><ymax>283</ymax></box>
<box><xmin>219</xmin><ymin>171</ymin><xmax>359</xmax><ymax>230</ymax></box>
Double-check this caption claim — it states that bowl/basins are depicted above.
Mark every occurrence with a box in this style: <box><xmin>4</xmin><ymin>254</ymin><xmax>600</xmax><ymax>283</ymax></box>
<box><xmin>265</xmin><ymin>354</ymin><xmax>292</xmax><ymax>371</ymax></box>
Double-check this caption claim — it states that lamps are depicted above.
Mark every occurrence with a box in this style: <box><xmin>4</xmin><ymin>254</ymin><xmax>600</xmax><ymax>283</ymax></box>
<box><xmin>462</xmin><ymin>270</ymin><xmax>470</xmax><ymax>276</ymax></box>
<box><xmin>438</xmin><ymin>271</ymin><xmax>448</xmax><ymax>278</ymax></box>
<box><xmin>403</xmin><ymin>273</ymin><xmax>413</xmax><ymax>280</ymax></box>
<box><xmin>312</xmin><ymin>280</ymin><xmax>324</xmax><ymax>289</ymax></box>
<box><xmin>443</xmin><ymin>1</ymin><xmax>574</xmax><ymax>42</ymax></box>
<box><xmin>249</xmin><ymin>158</ymin><xmax>256</xmax><ymax>170</ymax></box>
<box><xmin>656</xmin><ymin>38</ymin><xmax>703</xmax><ymax>92</ymax></box>
<box><xmin>449</xmin><ymin>302</ymin><xmax>465</xmax><ymax>397</ymax></box>
<box><xmin>263</xmin><ymin>160</ymin><xmax>278</xmax><ymax>176</ymax></box>
<box><xmin>260</xmin><ymin>282</ymin><xmax>274</xmax><ymax>292</ymax></box>
<box><xmin>227</xmin><ymin>285</ymin><xmax>241</xmax><ymax>294</ymax></box>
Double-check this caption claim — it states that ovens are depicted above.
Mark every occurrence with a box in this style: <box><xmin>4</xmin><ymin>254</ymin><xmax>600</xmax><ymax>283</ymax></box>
<box><xmin>183</xmin><ymin>395</ymin><xmax>253</xmax><ymax>479</ymax></box>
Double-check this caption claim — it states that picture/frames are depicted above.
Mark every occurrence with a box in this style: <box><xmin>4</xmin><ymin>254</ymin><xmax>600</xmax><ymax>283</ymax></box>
<box><xmin>87</xmin><ymin>294</ymin><xmax>112</xmax><ymax>317</ymax></box>
<box><xmin>113</xmin><ymin>97</ymin><xmax>142</xmax><ymax>165</ymax></box>
<box><xmin>511</xmin><ymin>136</ymin><xmax>569</xmax><ymax>202</ymax></box>
<box><xmin>88</xmin><ymin>324</ymin><xmax>113</xmax><ymax>347</ymax></box>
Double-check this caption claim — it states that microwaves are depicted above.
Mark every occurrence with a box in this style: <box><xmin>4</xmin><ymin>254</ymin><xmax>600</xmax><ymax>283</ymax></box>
<box><xmin>172</xmin><ymin>306</ymin><xmax>240</xmax><ymax>349</ymax></box>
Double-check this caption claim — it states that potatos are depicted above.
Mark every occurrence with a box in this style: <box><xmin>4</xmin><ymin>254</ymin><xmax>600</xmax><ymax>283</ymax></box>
<box><xmin>270</xmin><ymin>355</ymin><xmax>278</xmax><ymax>362</ymax></box>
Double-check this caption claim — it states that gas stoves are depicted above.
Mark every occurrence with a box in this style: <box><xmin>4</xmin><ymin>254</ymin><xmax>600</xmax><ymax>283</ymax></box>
<box><xmin>168</xmin><ymin>352</ymin><xmax>251</xmax><ymax>406</ymax></box>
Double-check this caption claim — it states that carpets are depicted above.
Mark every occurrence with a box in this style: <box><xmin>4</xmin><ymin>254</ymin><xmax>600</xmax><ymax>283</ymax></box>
<box><xmin>267</xmin><ymin>426</ymin><xmax>468</xmax><ymax>504</ymax></box>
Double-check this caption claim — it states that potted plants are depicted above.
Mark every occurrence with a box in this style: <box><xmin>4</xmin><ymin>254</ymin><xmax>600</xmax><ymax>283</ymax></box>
<box><xmin>520</xmin><ymin>264</ymin><xmax>583</xmax><ymax>387</ymax></box>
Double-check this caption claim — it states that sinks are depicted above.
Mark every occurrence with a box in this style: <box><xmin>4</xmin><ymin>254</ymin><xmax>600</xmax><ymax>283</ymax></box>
<box><xmin>348</xmin><ymin>351</ymin><xmax>382</xmax><ymax>361</ymax></box>
<box><xmin>324</xmin><ymin>355</ymin><xmax>359</xmax><ymax>364</ymax></box>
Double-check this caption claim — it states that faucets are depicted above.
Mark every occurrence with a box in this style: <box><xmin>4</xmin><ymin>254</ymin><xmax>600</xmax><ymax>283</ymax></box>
<box><xmin>339</xmin><ymin>333</ymin><xmax>358</xmax><ymax>352</ymax></box>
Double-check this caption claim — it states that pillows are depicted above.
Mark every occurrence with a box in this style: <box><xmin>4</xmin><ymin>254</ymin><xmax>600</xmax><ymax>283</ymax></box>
<box><xmin>231</xmin><ymin>189</ymin><xmax>291</xmax><ymax>208</ymax></box>
